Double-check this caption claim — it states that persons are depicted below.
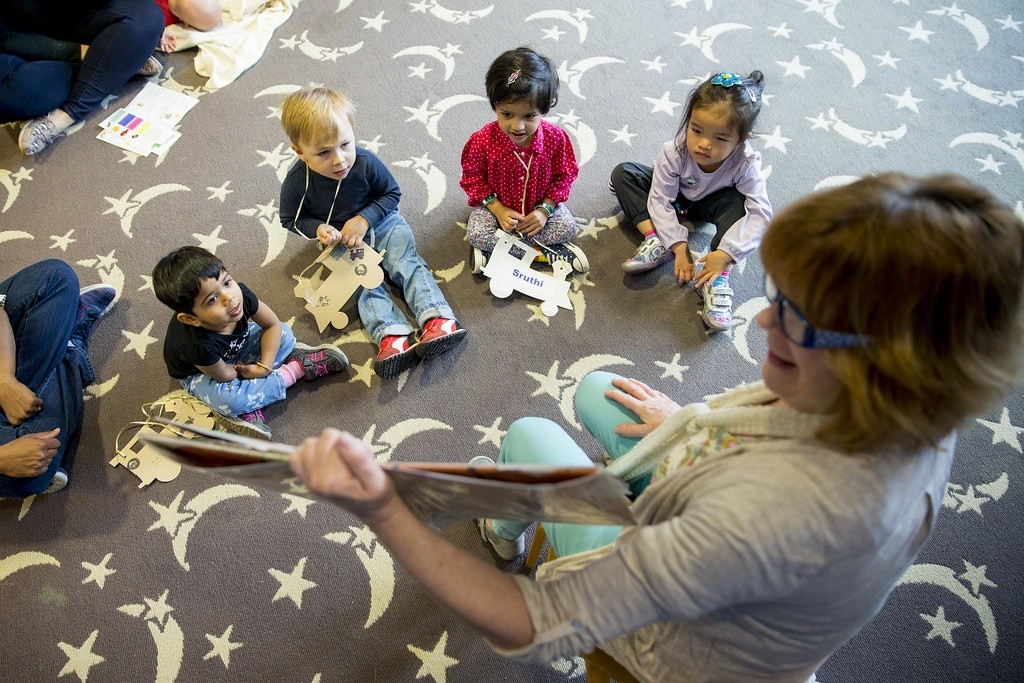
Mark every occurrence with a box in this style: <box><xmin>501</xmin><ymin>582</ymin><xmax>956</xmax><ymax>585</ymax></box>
<box><xmin>0</xmin><ymin>0</ymin><xmax>165</xmax><ymax>156</ymax></box>
<box><xmin>279</xmin><ymin>82</ymin><xmax>467</xmax><ymax>378</ymax></box>
<box><xmin>0</xmin><ymin>258</ymin><xmax>117</xmax><ymax>500</ymax></box>
<box><xmin>291</xmin><ymin>172</ymin><xmax>1024</xmax><ymax>683</ymax></box>
<box><xmin>153</xmin><ymin>0</ymin><xmax>222</xmax><ymax>54</ymax></box>
<box><xmin>460</xmin><ymin>47</ymin><xmax>590</xmax><ymax>274</ymax></box>
<box><xmin>152</xmin><ymin>245</ymin><xmax>349</xmax><ymax>441</ymax></box>
<box><xmin>609</xmin><ymin>70</ymin><xmax>772</xmax><ymax>332</ymax></box>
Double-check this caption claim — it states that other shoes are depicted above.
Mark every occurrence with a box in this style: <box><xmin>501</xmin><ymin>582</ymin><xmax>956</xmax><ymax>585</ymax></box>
<box><xmin>468</xmin><ymin>454</ymin><xmax>526</xmax><ymax>559</ymax></box>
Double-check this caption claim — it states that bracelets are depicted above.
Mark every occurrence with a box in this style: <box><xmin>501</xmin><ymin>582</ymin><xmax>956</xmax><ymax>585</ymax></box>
<box><xmin>481</xmin><ymin>193</ymin><xmax>498</xmax><ymax>208</ymax></box>
<box><xmin>257</xmin><ymin>362</ymin><xmax>273</xmax><ymax>372</ymax></box>
<box><xmin>534</xmin><ymin>203</ymin><xmax>553</xmax><ymax>217</ymax></box>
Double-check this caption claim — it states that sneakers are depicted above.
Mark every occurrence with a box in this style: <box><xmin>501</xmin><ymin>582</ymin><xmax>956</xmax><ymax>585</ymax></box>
<box><xmin>137</xmin><ymin>56</ymin><xmax>162</xmax><ymax>76</ymax></box>
<box><xmin>374</xmin><ymin>334</ymin><xmax>425</xmax><ymax>380</ymax></box>
<box><xmin>540</xmin><ymin>237</ymin><xmax>590</xmax><ymax>273</ymax></box>
<box><xmin>469</xmin><ymin>243</ymin><xmax>492</xmax><ymax>274</ymax></box>
<box><xmin>285</xmin><ymin>343</ymin><xmax>349</xmax><ymax>380</ymax></box>
<box><xmin>41</xmin><ymin>467</ymin><xmax>69</xmax><ymax>495</ymax></box>
<box><xmin>416</xmin><ymin>316</ymin><xmax>468</xmax><ymax>356</ymax></box>
<box><xmin>695</xmin><ymin>275</ymin><xmax>734</xmax><ymax>332</ymax></box>
<box><xmin>621</xmin><ymin>236</ymin><xmax>676</xmax><ymax>273</ymax></box>
<box><xmin>210</xmin><ymin>402</ymin><xmax>272</xmax><ymax>442</ymax></box>
<box><xmin>18</xmin><ymin>114</ymin><xmax>56</xmax><ymax>156</ymax></box>
<box><xmin>66</xmin><ymin>284</ymin><xmax>115</xmax><ymax>346</ymax></box>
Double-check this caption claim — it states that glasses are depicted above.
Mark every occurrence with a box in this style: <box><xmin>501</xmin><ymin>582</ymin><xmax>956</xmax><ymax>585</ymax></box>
<box><xmin>763</xmin><ymin>268</ymin><xmax>879</xmax><ymax>347</ymax></box>
